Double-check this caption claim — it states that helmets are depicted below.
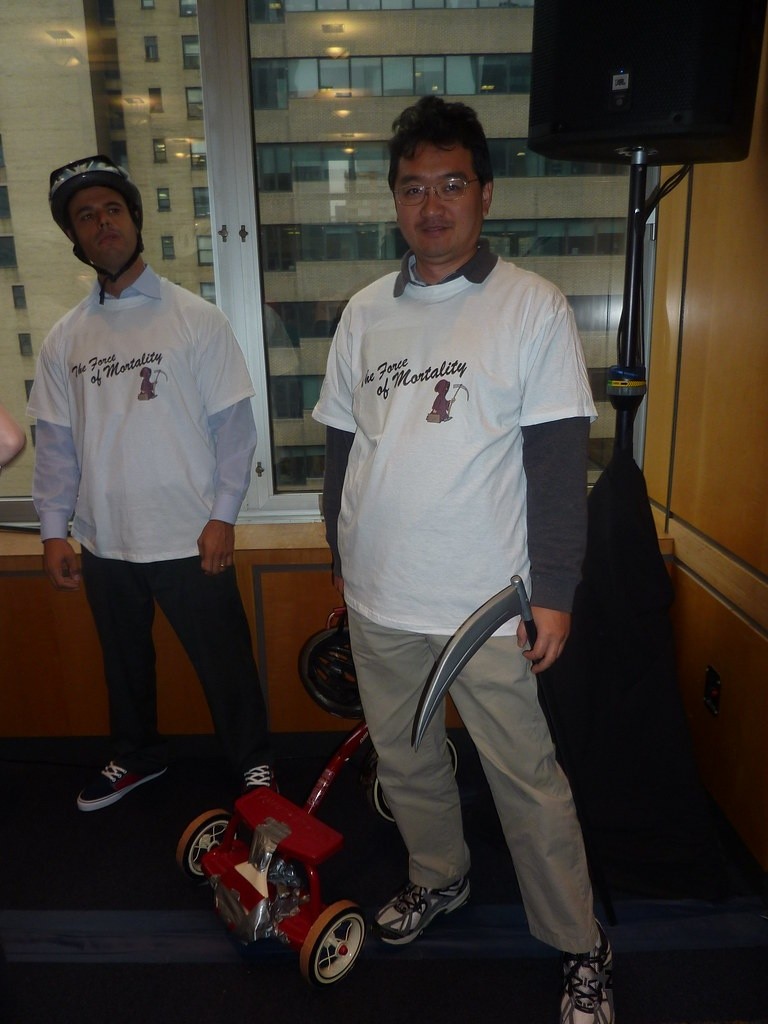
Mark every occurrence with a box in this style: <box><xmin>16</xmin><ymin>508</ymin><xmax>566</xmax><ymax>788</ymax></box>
<box><xmin>48</xmin><ymin>154</ymin><xmax>144</xmax><ymax>244</ymax></box>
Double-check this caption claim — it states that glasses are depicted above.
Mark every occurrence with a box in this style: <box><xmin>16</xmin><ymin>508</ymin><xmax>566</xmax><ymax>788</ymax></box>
<box><xmin>393</xmin><ymin>176</ymin><xmax>483</xmax><ymax>207</ymax></box>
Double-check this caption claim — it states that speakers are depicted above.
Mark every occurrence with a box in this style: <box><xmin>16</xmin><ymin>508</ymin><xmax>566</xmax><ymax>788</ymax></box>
<box><xmin>527</xmin><ymin>0</ymin><xmax>768</xmax><ymax>166</ymax></box>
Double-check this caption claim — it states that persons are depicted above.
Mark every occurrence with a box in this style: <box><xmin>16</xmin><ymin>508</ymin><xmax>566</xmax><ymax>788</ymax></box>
<box><xmin>313</xmin><ymin>93</ymin><xmax>619</xmax><ymax>1024</ymax></box>
<box><xmin>30</xmin><ymin>155</ymin><xmax>282</xmax><ymax>814</ymax></box>
<box><xmin>0</xmin><ymin>404</ymin><xmax>26</xmax><ymax>467</ymax></box>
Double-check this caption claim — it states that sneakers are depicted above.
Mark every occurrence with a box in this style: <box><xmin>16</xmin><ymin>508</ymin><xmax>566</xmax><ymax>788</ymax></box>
<box><xmin>371</xmin><ymin>875</ymin><xmax>473</xmax><ymax>946</ymax></box>
<box><xmin>77</xmin><ymin>762</ymin><xmax>170</xmax><ymax>811</ymax></box>
<box><xmin>557</xmin><ymin>918</ymin><xmax>614</xmax><ymax>1024</ymax></box>
<box><xmin>244</xmin><ymin>764</ymin><xmax>279</xmax><ymax>797</ymax></box>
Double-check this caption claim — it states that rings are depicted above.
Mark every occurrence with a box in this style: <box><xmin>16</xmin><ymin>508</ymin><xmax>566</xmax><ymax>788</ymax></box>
<box><xmin>220</xmin><ymin>564</ymin><xmax>227</xmax><ymax>568</ymax></box>
